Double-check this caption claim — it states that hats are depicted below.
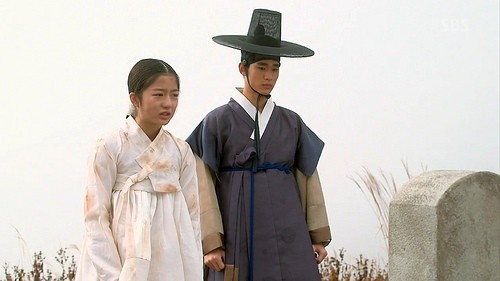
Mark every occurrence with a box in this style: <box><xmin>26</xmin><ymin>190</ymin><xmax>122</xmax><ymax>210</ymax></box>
<box><xmin>212</xmin><ymin>8</ymin><xmax>315</xmax><ymax>57</ymax></box>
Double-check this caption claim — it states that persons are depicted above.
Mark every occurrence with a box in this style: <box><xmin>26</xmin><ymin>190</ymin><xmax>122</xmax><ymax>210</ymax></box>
<box><xmin>184</xmin><ymin>9</ymin><xmax>332</xmax><ymax>281</ymax></box>
<box><xmin>74</xmin><ymin>58</ymin><xmax>205</xmax><ymax>281</ymax></box>
